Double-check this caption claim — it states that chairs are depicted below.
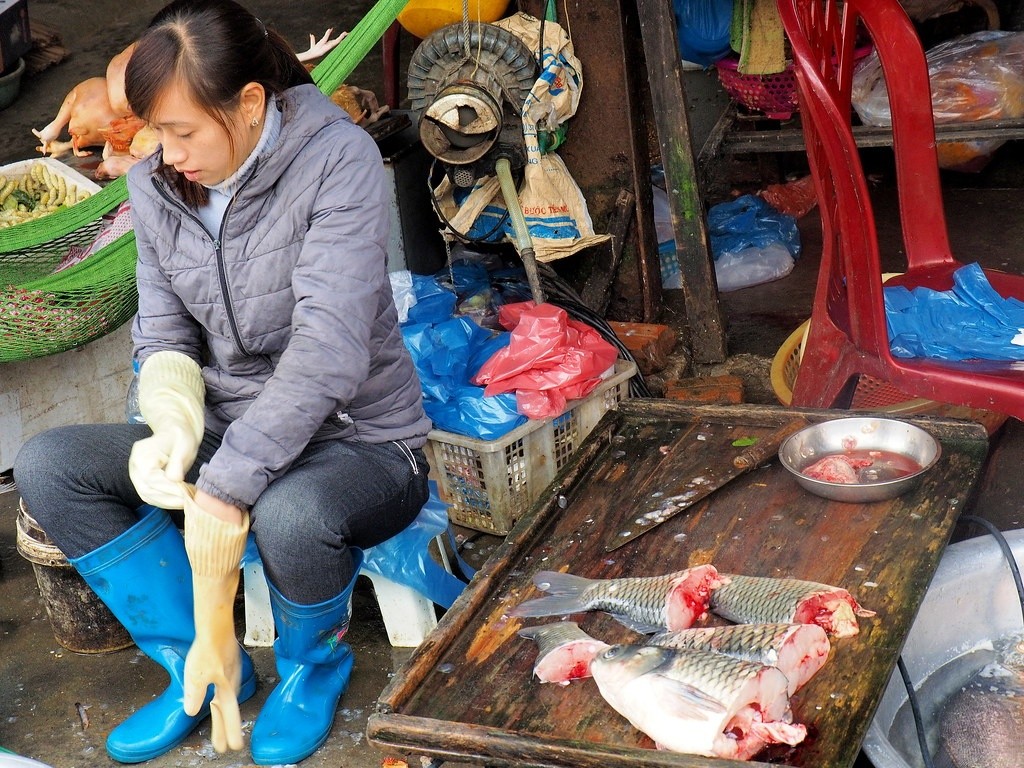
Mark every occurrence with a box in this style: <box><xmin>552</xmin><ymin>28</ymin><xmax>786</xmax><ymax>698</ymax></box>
<box><xmin>776</xmin><ymin>0</ymin><xmax>1024</xmax><ymax>427</ymax></box>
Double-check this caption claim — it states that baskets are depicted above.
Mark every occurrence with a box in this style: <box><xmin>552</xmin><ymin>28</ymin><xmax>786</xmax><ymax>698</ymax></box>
<box><xmin>715</xmin><ymin>40</ymin><xmax>873</xmax><ymax>111</ymax></box>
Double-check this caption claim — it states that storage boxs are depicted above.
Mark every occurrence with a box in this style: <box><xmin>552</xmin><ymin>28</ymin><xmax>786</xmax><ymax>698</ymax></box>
<box><xmin>421</xmin><ymin>358</ymin><xmax>639</xmax><ymax>537</ymax></box>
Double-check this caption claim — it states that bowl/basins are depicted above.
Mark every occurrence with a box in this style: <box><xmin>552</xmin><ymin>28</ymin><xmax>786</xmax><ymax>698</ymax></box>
<box><xmin>779</xmin><ymin>416</ymin><xmax>941</xmax><ymax>502</ymax></box>
<box><xmin>0</xmin><ymin>57</ymin><xmax>25</xmax><ymax>110</ymax></box>
<box><xmin>864</xmin><ymin>529</ymin><xmax>1024</xmax><ymax>767</ymax></box>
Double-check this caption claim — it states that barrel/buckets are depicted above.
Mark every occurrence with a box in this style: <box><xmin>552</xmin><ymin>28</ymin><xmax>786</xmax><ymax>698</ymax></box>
<box><xmin>16</xmin><ymin>497</ymin><xmax>135</xmax><ymax>653</ymax></box>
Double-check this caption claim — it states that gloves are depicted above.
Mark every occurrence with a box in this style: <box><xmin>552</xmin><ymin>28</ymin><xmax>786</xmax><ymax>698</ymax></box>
<box><xmin>179</xmin><ymin>481</ymin><xmax>250</xmax><ymax>753</ymax></box>
<box><xmin>128</xmin><ymin>351</ymin><xmax>205</xmax><ymax>509</ymax></box>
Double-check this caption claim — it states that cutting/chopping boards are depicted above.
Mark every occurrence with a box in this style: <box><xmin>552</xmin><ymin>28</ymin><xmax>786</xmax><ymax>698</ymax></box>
<box><xmin>366</xmin><ymin>399</ymin><xmax>989</xmax><ymax>767</ymax></box>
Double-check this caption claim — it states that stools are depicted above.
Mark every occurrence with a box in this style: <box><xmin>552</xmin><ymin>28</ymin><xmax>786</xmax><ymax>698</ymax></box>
<box><xmin>243</xmin><ymin>480</ymin><xmax>459</xmax><ymax>648</ymax></box>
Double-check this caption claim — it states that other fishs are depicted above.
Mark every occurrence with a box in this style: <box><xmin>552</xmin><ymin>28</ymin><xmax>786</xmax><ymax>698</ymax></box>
<box><xmin>513</xmin><ymin>564</ymin><xmax>877</xmax><ymax>764</ymax></box>
<box><xmin>939</xmin><ymin>629</ymin><xmax>1024</xmax><ymax>768</ymax></box>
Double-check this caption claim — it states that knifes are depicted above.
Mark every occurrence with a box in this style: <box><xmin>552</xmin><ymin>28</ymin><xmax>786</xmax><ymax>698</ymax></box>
<box><xmin>606</xmin><ymin>416</ymin><xmax>811</xmax><ymax>553</ymax></box>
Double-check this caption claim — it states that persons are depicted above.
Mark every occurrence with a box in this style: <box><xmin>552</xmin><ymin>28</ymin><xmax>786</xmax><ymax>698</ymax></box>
<box><xmin>12</xmin><ymin>1</ymin><xmax>431</xmax><ymax>765</ymax></box>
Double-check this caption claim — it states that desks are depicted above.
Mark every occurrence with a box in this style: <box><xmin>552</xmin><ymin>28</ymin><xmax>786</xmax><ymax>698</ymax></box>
<box><xmin>367</xmin><ymin>397</ymin><xmax>991</xmax><ymax>768</ymax></box>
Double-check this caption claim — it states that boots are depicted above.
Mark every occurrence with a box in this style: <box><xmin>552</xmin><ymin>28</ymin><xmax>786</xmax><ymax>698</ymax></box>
<box><xmin>251</xmin><ymin>547</ymin><xmax>364</xmax><ymax>765</ymax></box>
<box><xmin>67</xmin><ymin>504</ymin><xmax>257</xmax><ymax>762</ymax></box>
